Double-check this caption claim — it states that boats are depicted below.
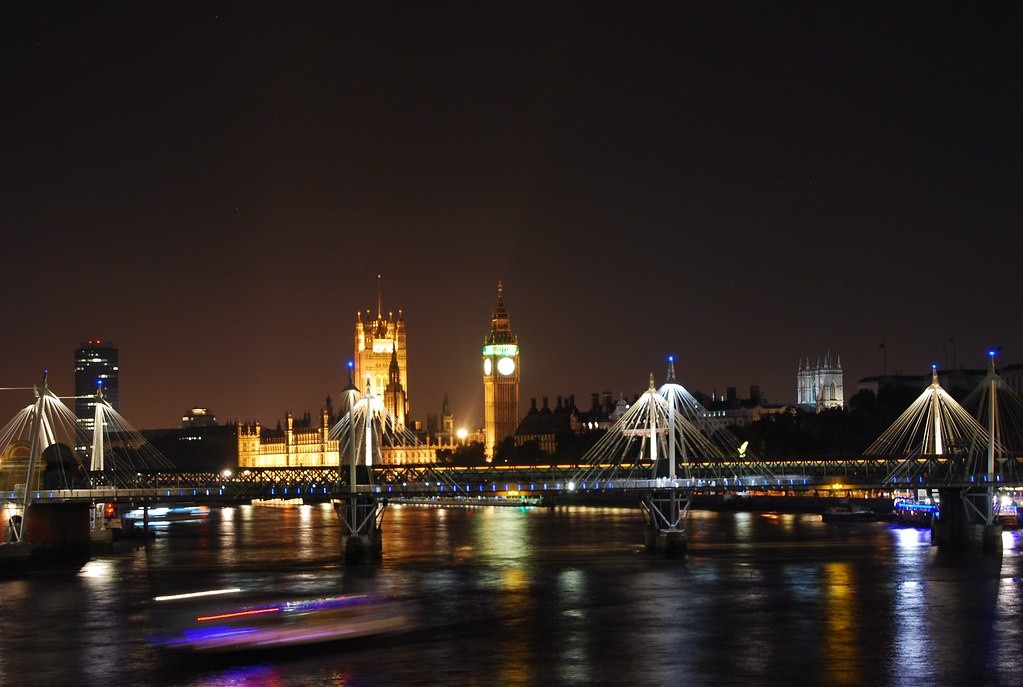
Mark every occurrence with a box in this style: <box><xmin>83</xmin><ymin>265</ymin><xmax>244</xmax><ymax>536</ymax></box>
<box><xmin>821</xmin><ymin>497</ymin><xmax>875</xmax><ymax>523</ymax></box>
